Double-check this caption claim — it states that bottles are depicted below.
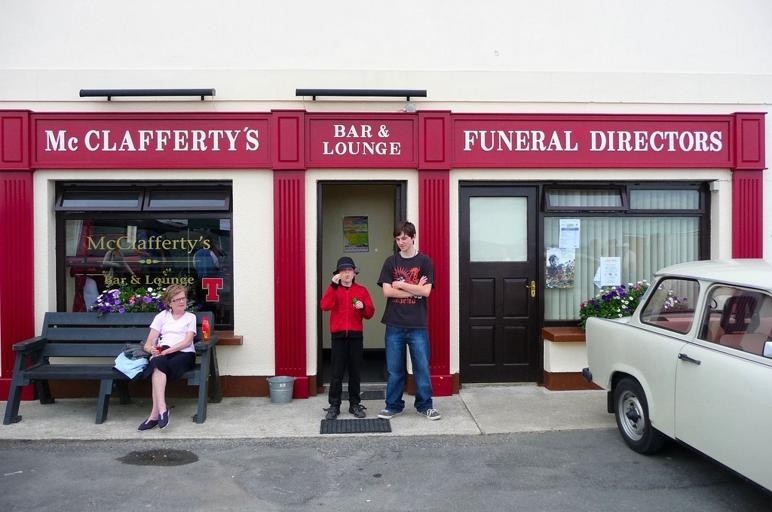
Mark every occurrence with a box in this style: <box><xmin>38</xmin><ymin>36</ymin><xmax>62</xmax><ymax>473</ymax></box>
<box><xmin>352</xmin><ymin>297</ymin><xmax>358</xmax><ymax>304</ymax></box>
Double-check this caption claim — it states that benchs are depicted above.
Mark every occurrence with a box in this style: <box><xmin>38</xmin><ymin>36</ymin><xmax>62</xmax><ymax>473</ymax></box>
<box><xmin>3</xmin><ymin>312</ymin><xmax>223</xmax><ymax>425</ymax></box>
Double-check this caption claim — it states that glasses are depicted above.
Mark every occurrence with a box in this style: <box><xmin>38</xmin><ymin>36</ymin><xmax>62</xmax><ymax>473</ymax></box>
<box><xmin>172</xmin><ymin>297</ymin><xmax>186</xmax><ymax>302</ymax></box>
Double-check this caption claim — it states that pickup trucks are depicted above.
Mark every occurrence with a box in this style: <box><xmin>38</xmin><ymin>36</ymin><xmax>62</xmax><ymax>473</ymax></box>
<box><xmin>568</xmin><ymin>258</ymin><xmax>772</xmax><ymax>493</ymax></box>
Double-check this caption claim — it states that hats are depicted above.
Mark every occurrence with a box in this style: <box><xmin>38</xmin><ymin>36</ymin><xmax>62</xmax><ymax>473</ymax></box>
<box><xmin>332</xmin><ymin>257</ymin><xmax>360</xmax><ymax>275</ymax></box>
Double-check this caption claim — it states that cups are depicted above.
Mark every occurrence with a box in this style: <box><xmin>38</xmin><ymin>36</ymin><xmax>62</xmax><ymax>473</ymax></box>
<box><xmin>151</xmin><ymin>337</ymin><xmax>163</xmax><ymax>354</ymax></box>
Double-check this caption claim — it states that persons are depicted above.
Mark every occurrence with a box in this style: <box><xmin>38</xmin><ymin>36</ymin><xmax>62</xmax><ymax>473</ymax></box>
<box><xmin>101</xmin><ymin>240</ymin><xmax>137</xmax><ymax>288</ymax></box>
<box><xmin>377</xmin><ymin>222</ymin><xmax>442</xmax><ymax>420</ymax></box>
<box><xmin>138</xmin><ymin>284</ymin><xmax>197</xmax><ymax>430</ymax></box>
<box><xmin>192</xmin><ymin>236</ymin><xmax>220</xmax><ymax>280</ymax></box>
<box><xmin>323</xmin><ymin>257</ymin><xmax>375</xmax><ymax>420</ymax></box>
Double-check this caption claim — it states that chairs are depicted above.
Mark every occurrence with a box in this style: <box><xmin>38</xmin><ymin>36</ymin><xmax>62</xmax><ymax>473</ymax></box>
<box><xmin>654</xmin><ymin>319</ymin><xmax>712</xmax><ymax>343</ymax></box>
<box><xmin>721</xmin><ymin>296</ymin><xmax>768</xmax><ymax>359</ymax></box>
<box><xmin>716</xmin><ymin>317</ymin><xmax>772</xmax><ymax>342</ymax></box>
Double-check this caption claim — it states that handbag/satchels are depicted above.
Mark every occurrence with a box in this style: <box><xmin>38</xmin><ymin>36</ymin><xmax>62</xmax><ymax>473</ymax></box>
<box><xmin>123</xmin><ymin>344</ymin><xmax>152</xmax><ymax>360</ymax></box>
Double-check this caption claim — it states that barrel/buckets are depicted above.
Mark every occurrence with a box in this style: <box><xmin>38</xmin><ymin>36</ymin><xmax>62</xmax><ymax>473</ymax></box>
<box><xmin>266</xmin><ymin>376</ymin><xmax>295</xmax><ymax>404</ymax></box>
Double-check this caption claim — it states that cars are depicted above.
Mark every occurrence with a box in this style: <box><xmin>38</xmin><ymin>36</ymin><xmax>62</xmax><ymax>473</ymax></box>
<box><xmin>67</xmin><ymin>234</ymin><xmax>157</xmax><ymax>281</ymax></box>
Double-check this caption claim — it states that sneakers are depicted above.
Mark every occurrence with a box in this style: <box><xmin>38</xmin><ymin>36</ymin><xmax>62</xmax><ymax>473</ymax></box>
<box><xmin>349</xmin><ymin>402</ymin><xmax>367</xmax><ymax>417</ymax></box>
<box><xmin>378</xmin><ymin>408</ymin><xmax>404</xmax><ymax>419</ymax></box>
<box><xmin>322</xmin><ymin>404</ymin><xmax>340</xmax><ymax>420</ymax></box>
<box><xmin>138</xmin><ymin>417</ymin><xmax>158</xmax><ymax>430</ymax></box>
<box><xmin>158</xmin><ymin>407</ymin><xmax>170</xmax><ymax>429</ymax></box>
<box><xmin>416</xmin><ymin>409</ymin><xmax>441</xmax><ymax>420</ymax></box>
